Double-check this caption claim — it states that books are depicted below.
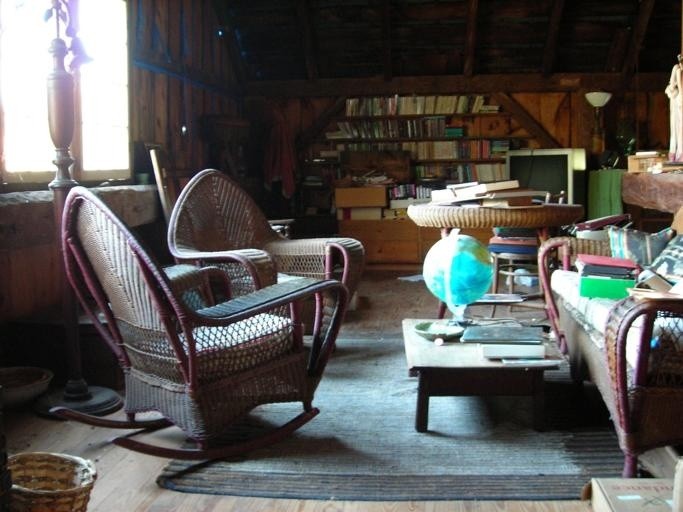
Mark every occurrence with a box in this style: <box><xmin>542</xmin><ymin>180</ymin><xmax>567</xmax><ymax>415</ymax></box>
<box><xmin>430</xmin><ymin>179</ymin><xmax>551</xmax><ymax>208</ymax></box>
<box><xmin>575</xmin><ymin>253</ymin><xmax>636</xmax><ymax>278</ymax></box>
<box><xmin>460</xmin><ymin>325</ymin><xmax>544</xmax><ymax>344</ymax></box>
<box><xmin>481</xmin><ymin>343</ymin><xmax>546</xmax><ymax>359</ymax></box>
<box><xmin>412</xmin><ymin>162</ymin><xmax>507</xmax><ymax>182</ymax></box>
<box><xmin>382</xmin><ymin>208</ymin><xmax>409</xmax><ymax>221</ymax></box>
<box><xmin>342</xmin><ymin>94</ymin><xmax>501</xmax><ymax>114</ymax></box>
<box><xmin>323</xmin><ymin>116</ymin><xmax>469</xmax><ymax>139</ymax></box>
<box><xmin>502</xmin><ymin>359</ymin><xmax>562</xmax><ymax>366</ymax></box>
<box><xmin>318</xmin><ymin>141</ymin><xmax>519</xmax><ymax>161</ymax></box>
<box><xmin>635</xmin><ymin>149</ymin><xmax>660</xmax><ymax>156</ymax></box>
<box><xmin>661</xmin><ymin>161</ymin><xmax>682</xmax><ymax>173</ymax></box>
<box><xmin>321</xmin><ymin>165</ymin><xmax>398</xmax><ymax>186</ymax></box>
<box><xmin>388</xmin><ymin>184</ymin><xmax>434</xmax><ymax>200</ymax></box>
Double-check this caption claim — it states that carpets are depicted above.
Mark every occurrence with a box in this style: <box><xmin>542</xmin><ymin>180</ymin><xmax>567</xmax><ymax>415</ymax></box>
<box><xmin>156</xmin><ymin>334</ymin><xmax>625</xmax><ymax>500</ymax></box>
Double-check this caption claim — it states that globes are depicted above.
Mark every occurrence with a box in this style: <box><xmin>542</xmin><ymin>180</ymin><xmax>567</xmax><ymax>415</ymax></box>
<box><xmin>422</xmin><ymin>234</ymin><xmax>494</xmax><ymax>327</ymax></box>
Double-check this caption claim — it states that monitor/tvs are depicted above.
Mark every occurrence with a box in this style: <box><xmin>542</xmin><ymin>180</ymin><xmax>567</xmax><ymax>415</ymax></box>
<box><xmin>506</xmin><ymin>149</ymin><xmax>574</xmax><ymax>207</ymax></box>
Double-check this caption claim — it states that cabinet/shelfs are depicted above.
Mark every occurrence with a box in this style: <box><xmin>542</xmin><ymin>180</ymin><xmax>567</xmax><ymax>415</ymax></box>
<box><xmin>293</xmin><ymin>92</ymin><xmax>563</xmax><ymax>263</ymax></box>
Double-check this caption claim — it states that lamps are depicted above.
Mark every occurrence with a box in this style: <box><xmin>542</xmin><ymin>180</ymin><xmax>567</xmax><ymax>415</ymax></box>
<box><xmin>585</xmin><ymin>92</ymin><xmax>612</xmax><ymax>156</ymax></box>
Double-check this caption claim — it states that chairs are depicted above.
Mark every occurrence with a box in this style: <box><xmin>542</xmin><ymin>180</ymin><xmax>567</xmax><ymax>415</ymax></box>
<box><xmin>50</xmin><ymin>185</ymin><xmax>348</xmax><ymax>461</ymax></box>
<box><xmin>538</xmin><ymin>204</ymin><xmax>682</xmax><ymax>478</ymax></box>
<box><xmin>489</xmin><ymin>190</ymin><xmax>566</xmax><ymax>318</ymax></box>
<box><xmin>167</xmin><ymin>169</ymin><xmax>366</xmax><ymax>353</ymax></box>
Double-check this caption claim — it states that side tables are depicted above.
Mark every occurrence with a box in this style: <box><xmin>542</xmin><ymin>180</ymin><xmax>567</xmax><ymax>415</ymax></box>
<box><xmin>407</xmin><ymin>203</ymin><xmax>585</xmax><ymax>318</ymax></box>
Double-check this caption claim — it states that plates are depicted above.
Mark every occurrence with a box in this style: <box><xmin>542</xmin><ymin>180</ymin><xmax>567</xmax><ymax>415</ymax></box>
<box><xmin>413</xmin><ymin>321</ymin><xmax>464</xmax><ymax>342</ymax></box>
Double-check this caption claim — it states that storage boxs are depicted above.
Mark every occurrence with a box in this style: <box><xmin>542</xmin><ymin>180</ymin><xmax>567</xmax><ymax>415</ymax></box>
<box><xmin>334</xmin><ymin>187</ymin><xmax>388</xmax><ymax>208</ymax></box>
<box><xmin>579</xmin><ymin>278</ymin><xmax>639</xmax><ymax>303</ymax></box>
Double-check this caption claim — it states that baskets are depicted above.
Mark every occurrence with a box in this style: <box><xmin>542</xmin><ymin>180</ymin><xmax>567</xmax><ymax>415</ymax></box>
<box><xmin>0</xmin><ymin>366</ymin><xmax>54</xmax><ymax>404</ymax></box>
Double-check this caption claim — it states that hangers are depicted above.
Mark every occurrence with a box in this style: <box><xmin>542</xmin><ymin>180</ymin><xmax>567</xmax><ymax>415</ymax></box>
<box><xmin>32</xmin><ymin>0</ymin><xmax>124</xmax><ymax>417</ymax></box>
